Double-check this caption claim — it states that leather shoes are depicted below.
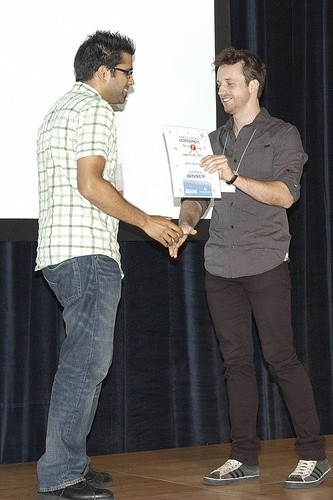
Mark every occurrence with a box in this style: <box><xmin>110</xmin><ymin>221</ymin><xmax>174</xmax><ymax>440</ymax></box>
<box><xmin>84</xmin><ymin>468</ymin><xmax>113</xmax><ymax>486</ymax></box>
<box><xmin>37</xmin><ymin>480</ymin><xmax>115</xmax><ymax>500</ymax></box>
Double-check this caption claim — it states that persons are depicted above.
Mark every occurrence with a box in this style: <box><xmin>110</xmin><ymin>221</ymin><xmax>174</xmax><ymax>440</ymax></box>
<box><xmin>169</xmin><ymin>47</ymin><xmax>332</xmax><ymax>489</ymax></box>
<box><xmin>34</xmin><ymin>29</ymin><xmax>184</xmax><ymax>500</ymax></box>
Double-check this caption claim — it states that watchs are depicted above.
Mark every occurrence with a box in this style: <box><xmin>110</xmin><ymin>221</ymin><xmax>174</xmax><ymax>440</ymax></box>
<box><xmin>226</xmin><ymin>170</ymin><xmax>239</xmax><ymax>185</ymax></box>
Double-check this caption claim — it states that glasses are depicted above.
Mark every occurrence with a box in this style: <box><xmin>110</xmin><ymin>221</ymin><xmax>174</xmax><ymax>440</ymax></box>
<box><xmin>113</xmin><ymin>66</ymin><xmax>133</xmax><ymax>75</ymax></box>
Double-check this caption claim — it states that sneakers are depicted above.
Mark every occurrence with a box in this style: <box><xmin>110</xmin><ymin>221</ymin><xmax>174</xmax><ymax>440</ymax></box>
<box><xmin>284</xmin><ymin>458</ymin><xmax>332</xmax><ymax>489</ymax></box>
<box><xmin>202</xmin><ymin>459</ymin><xmax>260</xmax><ymax>485</ymax></box>
